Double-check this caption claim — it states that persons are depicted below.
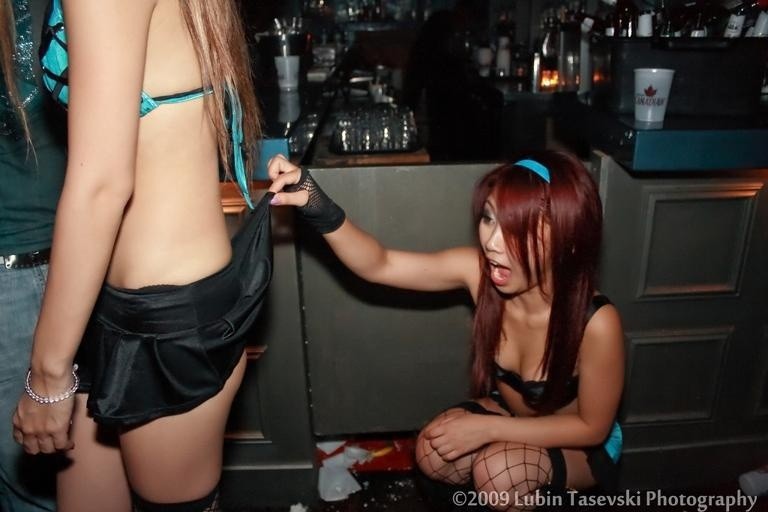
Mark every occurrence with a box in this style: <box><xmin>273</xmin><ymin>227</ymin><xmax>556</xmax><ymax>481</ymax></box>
<box><xmin>0</xmin><ymin>0</ymin><xmax>247</xmax><ymax>512</ymax></box>
<box><xmin>266</xmin><ymin>149</ymin><xmax>625</xmax><ymax>512</ymax></box>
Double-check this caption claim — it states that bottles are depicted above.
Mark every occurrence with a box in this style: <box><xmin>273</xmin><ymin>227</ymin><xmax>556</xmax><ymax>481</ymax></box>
<box><xmin>619</xmin><ymin>7</ymin><xmax>767</xmax><ymax>38</ymax></box>
<box><xmin>495</xmin><ymin>14</ymin><xmax>511</xmax><ymax>79</ymax></box>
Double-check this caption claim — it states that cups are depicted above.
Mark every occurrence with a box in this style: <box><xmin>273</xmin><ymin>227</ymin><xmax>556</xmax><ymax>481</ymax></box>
<box><xmin>275</xmin><ymin>55</ymin><xmax>299</xmax><ymax>91</ymax></box>
<box><xmin>333</xmin><ymin>104</ymin><xmax>418</xmax><ymax>152</ymax></box>
<box><xmin>633</xmin><ymin>68</ymin><xmax>675</xmax><ymax>123</ymax></box>
